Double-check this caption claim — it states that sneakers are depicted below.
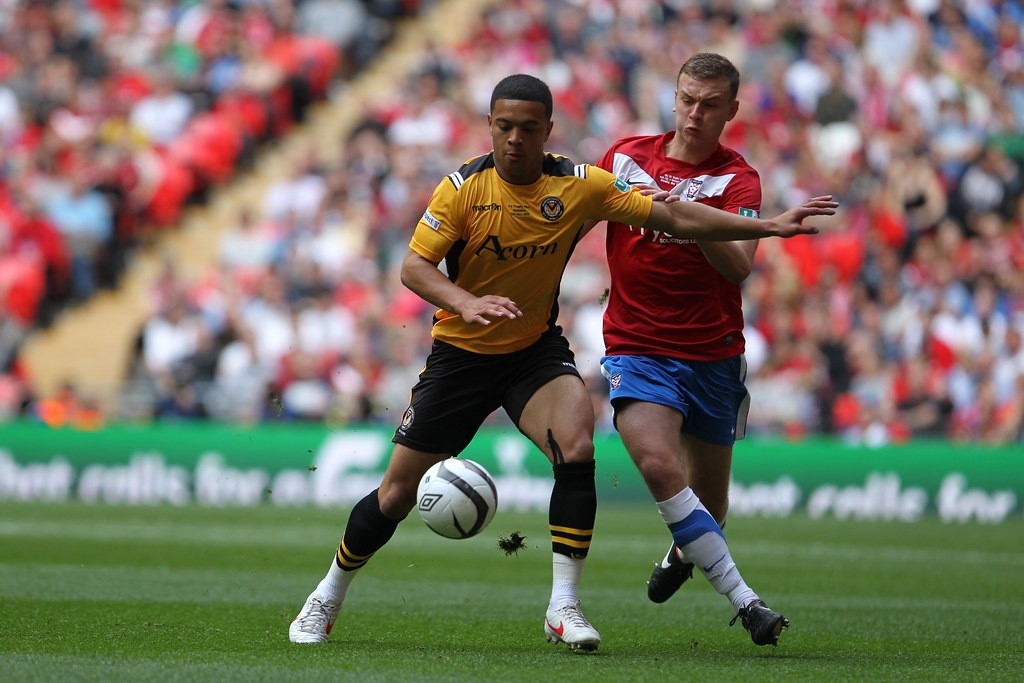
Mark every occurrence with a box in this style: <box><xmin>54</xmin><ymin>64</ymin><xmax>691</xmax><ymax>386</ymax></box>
<box><xmin>647</xmin><ymin>539</ymin><xmax>696</xmax><ymax>604</ymax></box>
<box><xmin>544</xmin><ymin>599</ymin><xmax>600</xmax><ymax>652</ymax></box>
<box><xmin>288</xmin><ymin>589</ymin><xmax>343</xmax><ymax>644</ymax></box>
<box><xmin>729</xmin><ymin>599</ymin><xmax>789</xmax><ymax>647</ymax></box>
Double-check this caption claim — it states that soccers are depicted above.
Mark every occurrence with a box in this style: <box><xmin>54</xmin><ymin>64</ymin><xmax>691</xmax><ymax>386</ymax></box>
<box><xmin>417</xmin><ymin>457</ymin><xmax>498</xmax><ymax>540</ymax></box>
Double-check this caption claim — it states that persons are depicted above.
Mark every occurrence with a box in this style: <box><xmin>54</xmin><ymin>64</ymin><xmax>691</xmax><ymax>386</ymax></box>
<box><xmin>1</xmin><ymin>0</ymin><xmax>1024</xmax><ymax>444</ymax></box>
<box><xmin>288</xmin><ymin>74</ymin><xmax>840</xmax><ymax>651</ymax></box>
<box><xmin>589</xmin><ymin>52</ymin><xmax>788</xmax><ymax>647</ymax></box>
